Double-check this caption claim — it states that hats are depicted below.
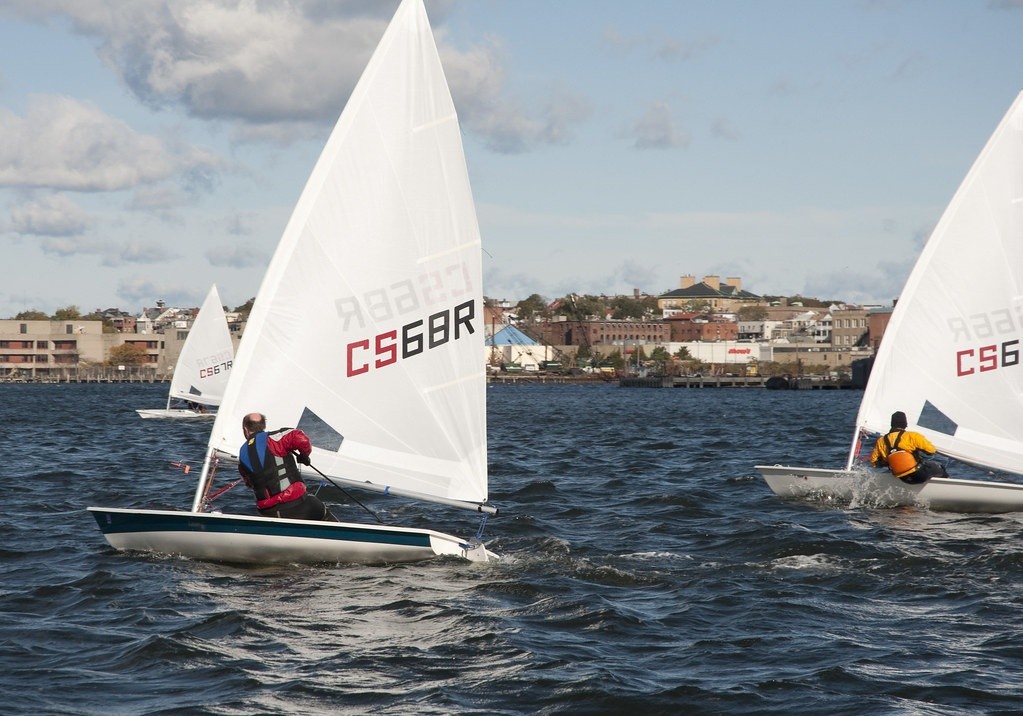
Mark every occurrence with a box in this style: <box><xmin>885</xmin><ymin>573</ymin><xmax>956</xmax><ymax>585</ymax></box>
<box><xmin>891</xmin><ymin>412</ymin><xmax>907</xmax><ymax>425</ymax></box>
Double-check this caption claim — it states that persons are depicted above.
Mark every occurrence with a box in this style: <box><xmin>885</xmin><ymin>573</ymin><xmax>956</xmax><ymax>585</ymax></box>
<box><xmin>871</xmin><ymin>411</ymin><xmax>950</xmax><ymax>484</ymax></box>
<box><xmin>238</xmin><ymin>413</ymin><xmax>325</xmax><ymax>519</ymax></box>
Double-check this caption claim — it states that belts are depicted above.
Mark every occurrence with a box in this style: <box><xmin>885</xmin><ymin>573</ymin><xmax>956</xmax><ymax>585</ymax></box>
<box><xmin>260</xmin><ymin>495</ymin><xmax>307</xmax><ymax>509</ymax></box>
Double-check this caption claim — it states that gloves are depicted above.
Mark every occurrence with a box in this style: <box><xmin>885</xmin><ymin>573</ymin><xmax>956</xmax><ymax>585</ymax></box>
<box><xmin>297</xmin><ymin>453</ymin><xmax>310</xmax><ymax>466</ymax></box>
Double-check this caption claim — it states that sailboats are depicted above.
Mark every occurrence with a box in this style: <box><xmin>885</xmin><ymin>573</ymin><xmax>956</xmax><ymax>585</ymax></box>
<box><xmin>754</xmin><ymin>89</ymin><xmax>1023</xmax><ymax>512</ymax></box>
<box><xmin>88</xmin><ymin>0</ymin><xmax>503</xmax><ymax>563</ymax></box>
